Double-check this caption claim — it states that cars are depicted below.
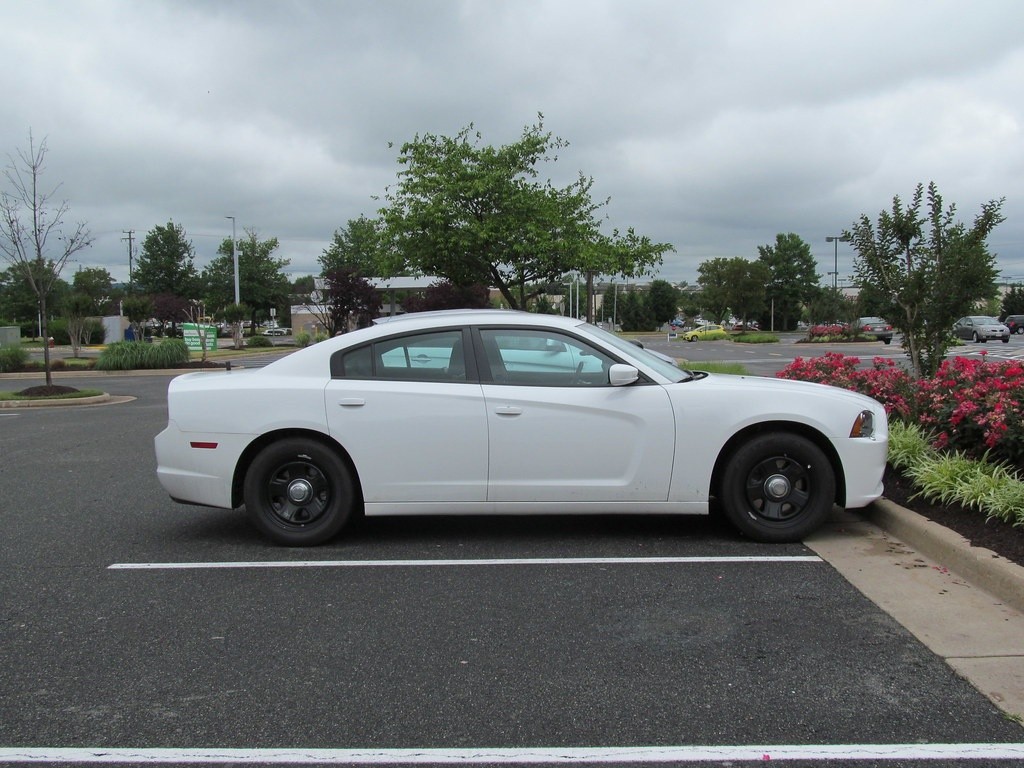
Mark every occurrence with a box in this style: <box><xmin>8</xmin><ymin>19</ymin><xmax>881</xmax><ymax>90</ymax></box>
<box><xmin>811</xmin><ymin>321</ymin><xmax>849</xmax><ymax>336</ymax></box>
<box><xmin>851</xmin><ymin>317</ymin><xmax>893</xmax><ymax>344</ymax></box>
<box><xmin>683</xmin><ymin>325</ymin><xmax>728</xmax><ymax>342</ymax></box>
<box><xmin>732</xmin><ymin>325</ymin><xmax>759</xmax><ymax>331</ymax></box>
<box><xmin>671</xmin><ymin>320</ymin><xmax>704</xmax><ymax>327</ymax></box>
<box><xmin>953</xmin><ymin>316</ymin><xmax>1011</xmax><ymax>344</ymax></box>
<box><xmin>141</xmin><ymin>318</ymin><xmax>260</xmax><ymax>339</ymax></box>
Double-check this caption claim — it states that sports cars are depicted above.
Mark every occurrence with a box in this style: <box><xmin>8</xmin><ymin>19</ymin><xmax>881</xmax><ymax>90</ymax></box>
<box><xmin>154</xmin><ymin>308</ymin><xmax>886</xmax><ymax>546</ymax></box>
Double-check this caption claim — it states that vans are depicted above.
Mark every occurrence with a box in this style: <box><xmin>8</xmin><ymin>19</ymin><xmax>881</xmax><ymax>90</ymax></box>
<box><xmin>369</xmin><ymin>309</ymin><xmax>678</xmax><ymax>372</ymax></box>
<box><xmin>263</xmin><ymin>328</ymin><xmax>292</xmax><ymax>336</ymax></box>
<box><xmin>1004</xmin><ymin>315</ymin><xmax>1024</xmax><ymax>335</ymax></box>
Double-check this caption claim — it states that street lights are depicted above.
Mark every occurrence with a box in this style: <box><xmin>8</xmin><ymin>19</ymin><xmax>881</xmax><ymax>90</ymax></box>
<box><xmin>826</xmin><ymin>236</ymin><xmax>846</xmax><ymax>288</ymax></box>
<box><xmin>225</xmin><ymin>216</ymin><xmax>241</xmax><ymax>306</ymax></box>
